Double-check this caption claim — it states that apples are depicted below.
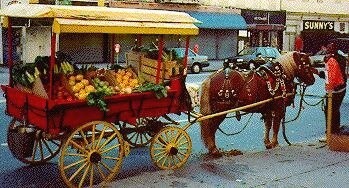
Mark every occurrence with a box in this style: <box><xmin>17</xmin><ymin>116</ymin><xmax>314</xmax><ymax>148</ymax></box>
<box><xmin>93</xmin><ymin>76</ymin><xmax>118</xmax><ymax>95</ymax></box>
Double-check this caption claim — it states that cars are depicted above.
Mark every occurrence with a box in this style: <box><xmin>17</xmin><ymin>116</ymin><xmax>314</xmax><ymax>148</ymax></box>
<box><xmin>223</xmin><ymin>46</ymin><xmax>283</xmax><ymax>70</ymax></box>
<box><xmin>166</xmin><ymin>48</ymin><xmax>210</xmax><ymax>74</ymax></box>
<box><xmin>311</xmin><ymin>50</ymin><xmax>349</xmax><ymax>66</ymax></box>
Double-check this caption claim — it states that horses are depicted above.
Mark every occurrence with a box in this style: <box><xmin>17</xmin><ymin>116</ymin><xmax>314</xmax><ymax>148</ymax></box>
<box><xmin>199</xmin><ymin>51</ymin><xmax>315</xmax><ymax>158</ymax></box>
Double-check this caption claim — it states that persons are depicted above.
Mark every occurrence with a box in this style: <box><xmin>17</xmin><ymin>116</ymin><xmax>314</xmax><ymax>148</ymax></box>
<box><xmin>318</xmin><ymin>42</ymin><xmax>347</xmax><ymax>141</ymax></box>
<box><xmin>311</xmin><ymin>40</ymin><xmax>348</xmax><ymax>133</ymax></box>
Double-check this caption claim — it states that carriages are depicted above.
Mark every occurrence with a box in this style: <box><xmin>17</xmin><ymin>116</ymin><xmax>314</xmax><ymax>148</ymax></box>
<box><xmin>0</xmin><ymin>3</ymin><xmax>315</xmax><ymax>188</ymax></box>
